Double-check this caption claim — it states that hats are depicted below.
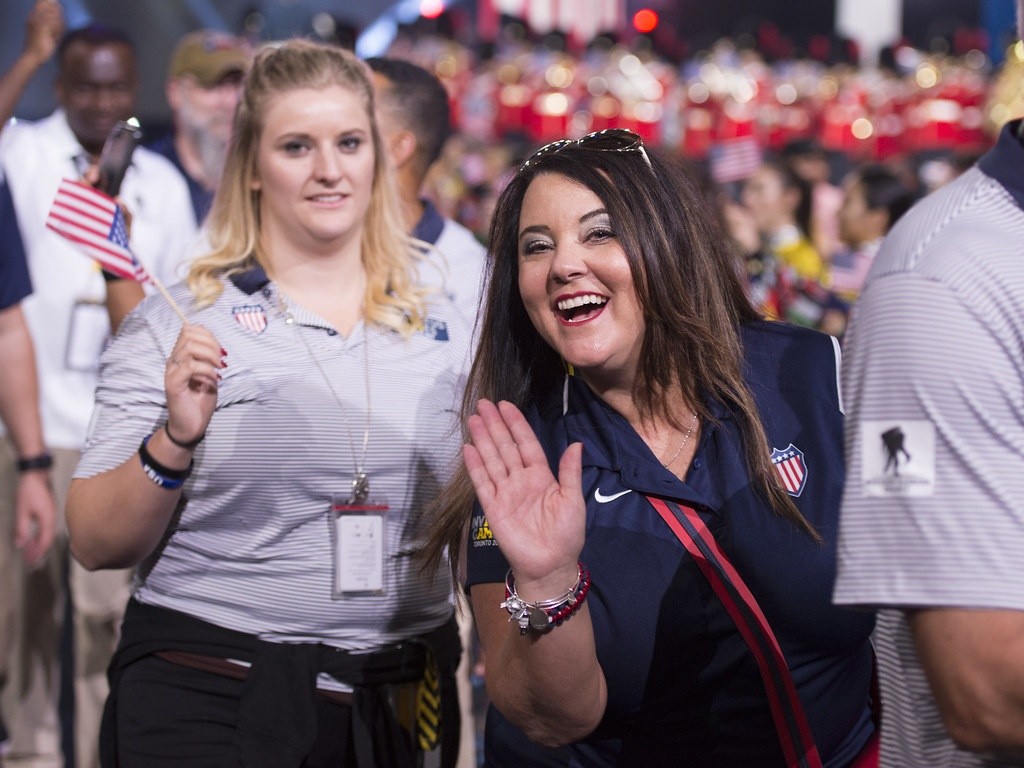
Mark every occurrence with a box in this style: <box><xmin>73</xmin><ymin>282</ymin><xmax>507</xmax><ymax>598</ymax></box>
<box><xmin>169</xmin><ymin>29</ymin><xmax>252</xmax><ymax>90</ymax></box>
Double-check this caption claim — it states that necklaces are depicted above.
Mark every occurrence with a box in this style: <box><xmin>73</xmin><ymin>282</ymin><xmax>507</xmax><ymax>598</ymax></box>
<box><xmin>663</xmin><ymin>410</ymin><xmax>698</xmax><ymax>469</ymax></box>
<box><xmin>276</xmin><ymin>278</ymin><xmax>371</xmax><ymax>501</ymax></box>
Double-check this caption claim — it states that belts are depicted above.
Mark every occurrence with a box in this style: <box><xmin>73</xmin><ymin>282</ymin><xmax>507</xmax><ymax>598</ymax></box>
<box><xmin>152</xmin><ymin>650</ymin><xmax>355</xmax><ymax>705</ymax></box>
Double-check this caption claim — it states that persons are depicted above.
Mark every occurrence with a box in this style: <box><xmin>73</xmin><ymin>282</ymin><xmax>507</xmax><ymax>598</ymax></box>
<box><xmin>832</xmin><ymin>113</ymin><xmax>1024</xmax><ymax>768</ymax></box>
<box><xmin>1</xmin><ymin>174</ymin><xmax>60</xmax><ymax>575</ymax></box>
<box><xmin>419</xmin><ymin>129</ymin><xmax>880</xmax><ymax>768</ymax></box>
<box><xmin>364</xmin><ymin>56</ymin><xmax>499</xmax><ymax>768</ymax></box>
<box><xmin>62</xmin><ymin>37</ymin><xmax>484</xmax><ymax>768</ymax></box>
<box><xmin>0</xmin><ymin>0</ymin><xmax>199</xmax><ymax>711</ymax></box>
<box><xmin>2</xmin><ymin>2</ymin><xmax>1024</xmax><ymax>344</ymax></box>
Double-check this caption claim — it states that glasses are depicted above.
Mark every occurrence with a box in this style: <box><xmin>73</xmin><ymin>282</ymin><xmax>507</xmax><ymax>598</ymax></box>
<box><xmin>519</xmin><ymin>128</ymin><xmax>659</xmax><ymax>179</ymax></box>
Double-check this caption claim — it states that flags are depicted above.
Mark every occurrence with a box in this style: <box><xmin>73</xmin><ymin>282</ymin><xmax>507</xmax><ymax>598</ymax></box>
<box><xmin>46</xmin><ymin>177</ymin><xmax>156</xmax><ymax>286</ymax></box>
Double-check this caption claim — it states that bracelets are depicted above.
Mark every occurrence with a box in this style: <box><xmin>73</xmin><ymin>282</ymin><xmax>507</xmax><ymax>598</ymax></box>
<box><xmin>500</xmin><ymin>559</ymin><xmax>591</xmax><ymax>636</ymax></box>
<box><xmin>137</xmin><ymin>434</ymin><xmax>194</xmax><ymax>490</ymax></box>
<box><xmin>164</xmin><ymin>420</ymin><xmax>205</xmax><ymax>448</ymax></box>
<box><xmin>16</xmin><ymin>452</ymin><xmax>51</xmax><ymax>472</ymax></box>
